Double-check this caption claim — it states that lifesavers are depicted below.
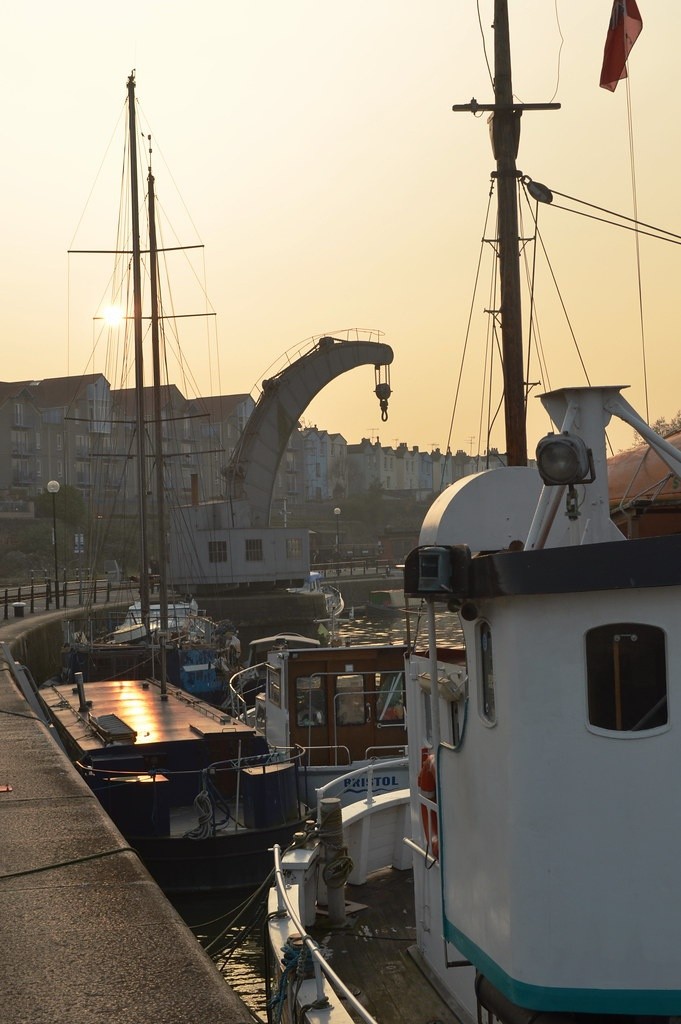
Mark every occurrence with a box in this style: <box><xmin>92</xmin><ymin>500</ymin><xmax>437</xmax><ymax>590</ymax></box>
<box><xmin>418</xmin><ymin>754</ymin><xmax>440</xmax><ymax>858</ymax></box>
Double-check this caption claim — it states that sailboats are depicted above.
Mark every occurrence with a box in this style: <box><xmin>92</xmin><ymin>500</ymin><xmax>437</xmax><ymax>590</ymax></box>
<box><xmin>59</xmin><ymin>0</ymin><xmax>681</xmax><ymax>1023</ymax></box>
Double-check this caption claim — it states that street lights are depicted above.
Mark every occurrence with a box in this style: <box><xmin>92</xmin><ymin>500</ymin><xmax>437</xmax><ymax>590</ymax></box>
<box><xmin>333</xmin><ymin>507</ymin><xmax>341</xmax><ymax>562</ymax></box>
<box><xmin>48</xmin><ymin>480</ymin><xmax>62</xmax><ymax>610</ymax></box>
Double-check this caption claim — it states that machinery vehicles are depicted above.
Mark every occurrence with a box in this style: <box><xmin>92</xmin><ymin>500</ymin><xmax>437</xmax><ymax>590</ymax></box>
<box><xmin>155</xmin><ymin>327</ymin><xmax>399</xmax><ymax>594</ymax></box>
